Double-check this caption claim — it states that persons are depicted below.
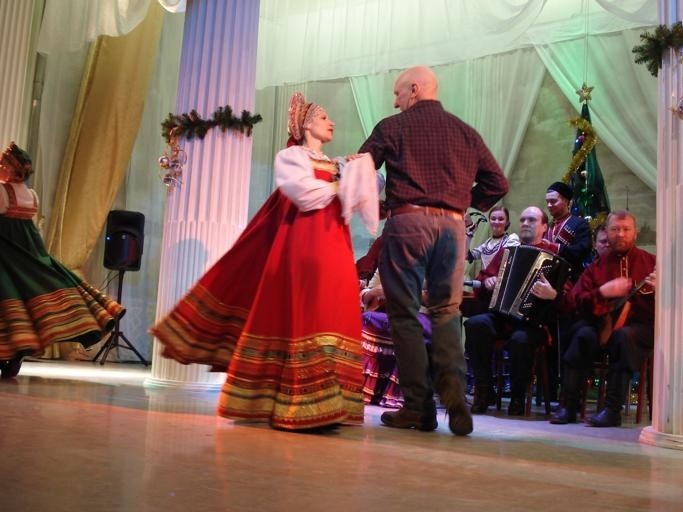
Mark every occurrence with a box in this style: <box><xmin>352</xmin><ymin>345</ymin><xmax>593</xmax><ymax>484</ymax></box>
<box><xmin>353</xmin><ymin>63</ymin><xmax>510</xmax><ymax>439</ymax></box>
<box><xmin>353</xmin><ymin>179</ymin><xmax>658</xmax><ymax>429</ymax></box>
<box><xmin>0</xmin><ymin>138</ymin><xmax>42</xmax><ymax>380</ymax></box>
<box><xmin>270</xmin><ymin>90</ymin><xmax>386</xmax><ymax>433</ymax></box>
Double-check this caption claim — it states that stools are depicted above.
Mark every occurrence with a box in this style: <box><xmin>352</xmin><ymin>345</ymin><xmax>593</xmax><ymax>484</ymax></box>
<box><xmin>493</xmin><ymin>336</ymin><xmax>653</xmax><ymax>423</ymax></box>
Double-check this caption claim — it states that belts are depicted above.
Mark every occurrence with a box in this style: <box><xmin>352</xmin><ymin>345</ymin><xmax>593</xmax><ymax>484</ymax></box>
<box><xmin>391</xmin><ymin>203</ymin><xmax>464</xmax><ymax>221</ymax></box>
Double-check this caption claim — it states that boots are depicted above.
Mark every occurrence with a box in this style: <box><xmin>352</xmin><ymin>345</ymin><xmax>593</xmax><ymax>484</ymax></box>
<box><xmin>587</xmin><ymin>383</ymin><xmax>628</xmax><ymax>426</ymax></box>
<box><xmin>550</xmin><ymin>374</ymin><xmax>584</xmax><ymax>424</ymax></box>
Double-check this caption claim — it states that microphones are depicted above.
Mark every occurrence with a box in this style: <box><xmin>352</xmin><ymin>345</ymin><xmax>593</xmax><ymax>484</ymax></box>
<box><xmin>463</xmin><ymin>279</ymin><xmax>482</xmax><ymax>288</ymax></box>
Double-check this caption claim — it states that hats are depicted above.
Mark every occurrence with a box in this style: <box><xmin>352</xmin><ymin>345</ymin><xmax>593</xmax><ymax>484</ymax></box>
<box><xmin>287</xmin><ymin>92</ymin><xmax>325</xmax><ymax>140</ymax></box>
<box><xmin>547</xmin><ymin>182</ymin><xmax>574</xmax><ymax>201</ymax></box>
<box><xmin>3</xmin><ymin>141</ymin><xmax>33</xmax><ymax>180</ymax></box>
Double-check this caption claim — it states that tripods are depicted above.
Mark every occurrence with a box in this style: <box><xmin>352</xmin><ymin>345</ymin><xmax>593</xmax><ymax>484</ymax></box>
<box><xmin>91</xmin><ymin>270</ymin><xmax>149</xmax><ymax>367</ymax></box>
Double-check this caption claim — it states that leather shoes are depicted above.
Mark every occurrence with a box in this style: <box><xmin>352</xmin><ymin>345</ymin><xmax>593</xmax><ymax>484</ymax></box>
<box><xmin>434</xmin><ymin>370</ymin><xmax>473</xmax><ymax>435</ymax></box>
<box><xmin>471</xmin><ymin>385</ymin><xmax>501</xmax><ymax>412</ymax></box>
<box><xmin>509</xmin><ymin>390</ymin><xmax>525</xmax><ymax>416</ymax></box>
<box><xmin>381</xmin><ymin>400</ymin><xmax>438</xmax><ymax>431</ymax></box>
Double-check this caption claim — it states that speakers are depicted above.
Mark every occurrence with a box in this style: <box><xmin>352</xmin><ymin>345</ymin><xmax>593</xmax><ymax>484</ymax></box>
<box><xmin>103</xmin><ymin>210</ymin><xmax>145</xmax><ymax>271</ymax></box>
